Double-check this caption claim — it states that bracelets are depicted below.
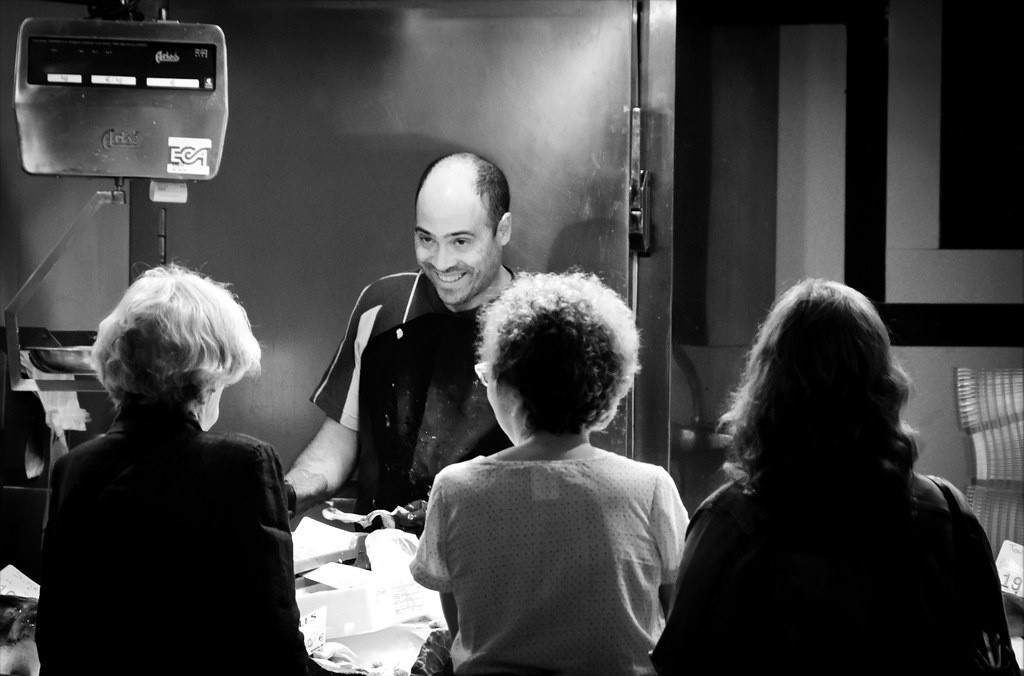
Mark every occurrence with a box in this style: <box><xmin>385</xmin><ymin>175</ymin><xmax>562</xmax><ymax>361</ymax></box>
<box><xmin>284</xmin><ymin>479</ymin><xmax>297</xmax><ymax>521</ymax></box>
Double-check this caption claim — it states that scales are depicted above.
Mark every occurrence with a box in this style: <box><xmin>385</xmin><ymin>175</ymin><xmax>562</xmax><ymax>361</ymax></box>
<box><xmin>1</xmin><ymin>14</ymin><xmax>231</xmax><ymax>394</ymax></box>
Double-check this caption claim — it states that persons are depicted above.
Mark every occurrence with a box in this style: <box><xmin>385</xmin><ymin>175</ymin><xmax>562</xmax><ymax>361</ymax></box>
<box><xmin>34</xmin><ymin>262</ymin><xmax>360</xmax><ymax>676</ymax></box>
<box><xmin>284</xmin><ymin>152</ymin><xmax>522</xmax><ymax>540</ymax></box>
<box><xmin>410</xmin><ymin>267</ymin><xmax>690</xmax><ymax>676</ymax></box>
<box><xmin>649</xmin><ymin>278</ymin><xmax>1022</xmax><ymax>676</ymax></box>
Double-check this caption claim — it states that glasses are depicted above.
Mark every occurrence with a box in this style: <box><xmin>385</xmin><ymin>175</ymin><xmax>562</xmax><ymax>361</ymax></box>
<box><xmin>475</xmin><ymin>362</ymin><xmax>492</xmax><ymax>387</ymax></box>
